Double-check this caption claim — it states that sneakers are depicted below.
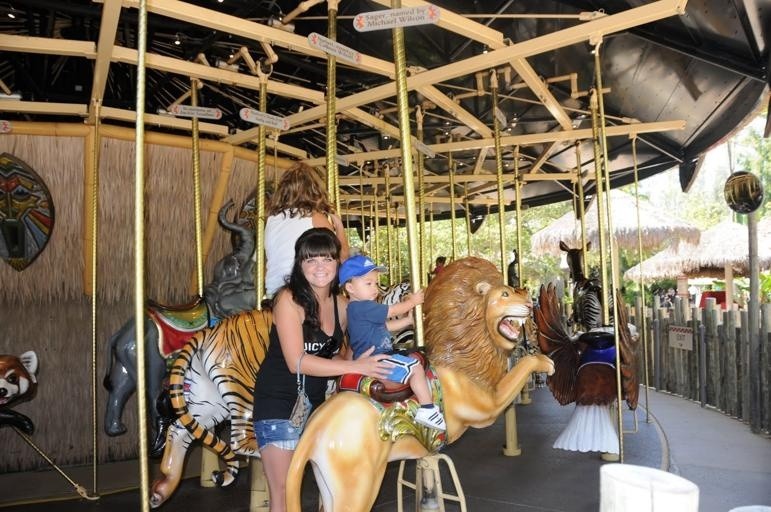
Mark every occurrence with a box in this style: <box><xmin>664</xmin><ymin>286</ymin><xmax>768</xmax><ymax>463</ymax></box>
<box><xmin>415</xmin><ymin>405</ymin><xmax>446</xmax><ymax>431</ymax></box>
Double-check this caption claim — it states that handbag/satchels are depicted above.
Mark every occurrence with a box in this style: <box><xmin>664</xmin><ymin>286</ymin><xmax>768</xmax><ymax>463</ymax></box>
<box><xmin>287</xmin><ymin>352</ymin><xmax>312</xmax><ymax>434</ymax></box>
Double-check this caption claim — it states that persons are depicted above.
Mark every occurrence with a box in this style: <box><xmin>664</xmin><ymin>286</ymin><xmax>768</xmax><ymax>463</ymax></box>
<box><xmin>652</xmin><ymin>286</ymin><xmax>676</xmax><ymax>308</ymax></box>
<box><xmin>430</xmin><ymin>257</ymin><xmax>447</xmax><ymax>275</ymax></box>
<box><xmin>338</xmin><ymin>255</ymin><xmax>447</xmax><ymax>431</ymax></box>
<box><xmin>252</xmin><ymin>228</ymin><xmax>396</xmax><ymax>511</ymax></box>
<box><xmin>262</xmin><ymin>162</ymin><xmax>352</xmax><ymax>302</ymax></box>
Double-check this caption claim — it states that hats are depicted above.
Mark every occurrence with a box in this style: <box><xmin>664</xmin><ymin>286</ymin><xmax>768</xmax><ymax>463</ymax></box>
<box><xmin>338</xmin><ymin>255</ymin><xmax>385</xmax><ymax>288</ymax></box>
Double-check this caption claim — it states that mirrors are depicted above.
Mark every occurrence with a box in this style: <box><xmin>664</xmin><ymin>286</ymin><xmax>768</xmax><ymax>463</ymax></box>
<box><xmin>724</xmin><ymin>170</ymin><xmax>764</xmax><ymax>215</ymax></box>
